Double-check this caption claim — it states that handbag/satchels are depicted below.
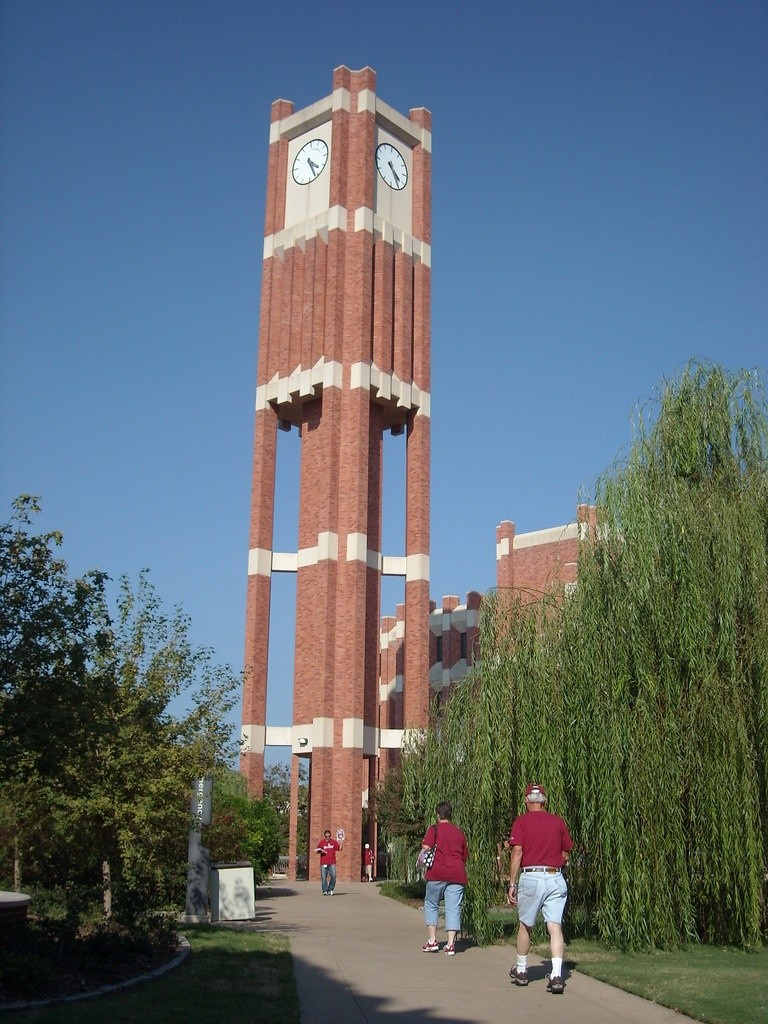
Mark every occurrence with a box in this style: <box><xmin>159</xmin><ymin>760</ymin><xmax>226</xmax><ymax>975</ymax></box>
<box><xmin>415</xmin><ymin>824</ymin><xmax>438</xmax><ymax>869</ymax></box>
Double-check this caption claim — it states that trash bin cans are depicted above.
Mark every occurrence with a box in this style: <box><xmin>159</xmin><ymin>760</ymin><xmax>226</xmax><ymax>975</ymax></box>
<box><xmin>210</xmin><ymin>861</ymin><xmax>252</xmax><ymax>922</ymax></box>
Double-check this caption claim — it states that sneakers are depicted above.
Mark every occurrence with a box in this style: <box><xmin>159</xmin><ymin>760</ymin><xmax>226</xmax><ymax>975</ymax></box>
<box><xmin>443</xmin><ymin>944</ymin><xmax>455</xmax><ymax>955</ymax></box>
<box><xmin>323</xmin><ymin>891</ymin><xmax>327</xmax><ymax>895</ymax></box>
<box><xmin>328</xmin><ymin>890</ymin><xmax>334</xmax><ymax>895</ymax></box>
<box><xmin>509</xmin><ymin>963</ymin><xmax>529</xmax><ymax>985</ymax></box>
<box><xmin>422</xmin><ymin>938</ymin><xmax>439</xmax><ymax>952</ymax></box>
<box><xmin>545</xmin><ymin>973</ymin><xmax>566</xmax><ymax>994</ymax></box>
<box><xmin>369</xmin><ymin>878</ymin><xmax>373</xmax><ymax>881</ymax></box>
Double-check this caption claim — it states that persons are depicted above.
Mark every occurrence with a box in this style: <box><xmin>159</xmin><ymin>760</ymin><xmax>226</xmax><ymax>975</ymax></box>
<box><xmin>507</xmin><ymin>784</ymin><xmax>574</xmax><ymax>995</ymax></box>
<box><xmin>421</xmin><ymin>801</ymin><xmax>469</xmax><ymax>956</ymax></box>
<box><xmin>362</xmin><ymin>843</ymin><xmax>375</xmax><ymax>882</ymax></box>
<box><xmin>317</xmin><ymin>830</ymin><xmax>343</xmax><ymax>896</ymax></box>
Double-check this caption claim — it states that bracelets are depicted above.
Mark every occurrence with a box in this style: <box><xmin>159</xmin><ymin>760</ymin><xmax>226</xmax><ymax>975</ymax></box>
<box><xmin>509</xmin><ymin>884</ymin><xmax>517</xmax><ymax>888</ymax></box>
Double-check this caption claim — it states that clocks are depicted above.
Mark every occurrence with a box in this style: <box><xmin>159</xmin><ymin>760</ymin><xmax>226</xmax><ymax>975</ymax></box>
<box><xmin>292</xmin><ymin>139</ymin><xmax>329</xmax><ymax>186</ymax></box>
<box><xmin>374</xmin><ymin>143</ymin><xmax>408</xmax><ymax>190</ymax></box>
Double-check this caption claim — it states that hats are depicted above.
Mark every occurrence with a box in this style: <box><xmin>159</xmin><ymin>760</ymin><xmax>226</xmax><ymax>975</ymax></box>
<box><xmin>526</xmin><ymin>784</ymin><xmax>546</xmax><ymax>797</ymax></box>
<box><xmin>365</xmin><ymin>844</ymin><xmax>369</xmax><ymax>849</ymax></box>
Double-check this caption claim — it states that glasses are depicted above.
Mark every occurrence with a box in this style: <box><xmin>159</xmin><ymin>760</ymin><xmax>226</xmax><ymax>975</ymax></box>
<box><xmin>325</xmin><ymin>835</ymin><xmax>330</xmax><ymax>838</ymax></box>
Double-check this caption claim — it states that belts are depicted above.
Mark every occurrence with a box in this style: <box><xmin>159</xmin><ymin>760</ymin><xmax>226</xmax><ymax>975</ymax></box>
<box><xmin>521</xmin><ymin>867</ymin><xmax>560</xmax><ymax>872</ymax></box>
